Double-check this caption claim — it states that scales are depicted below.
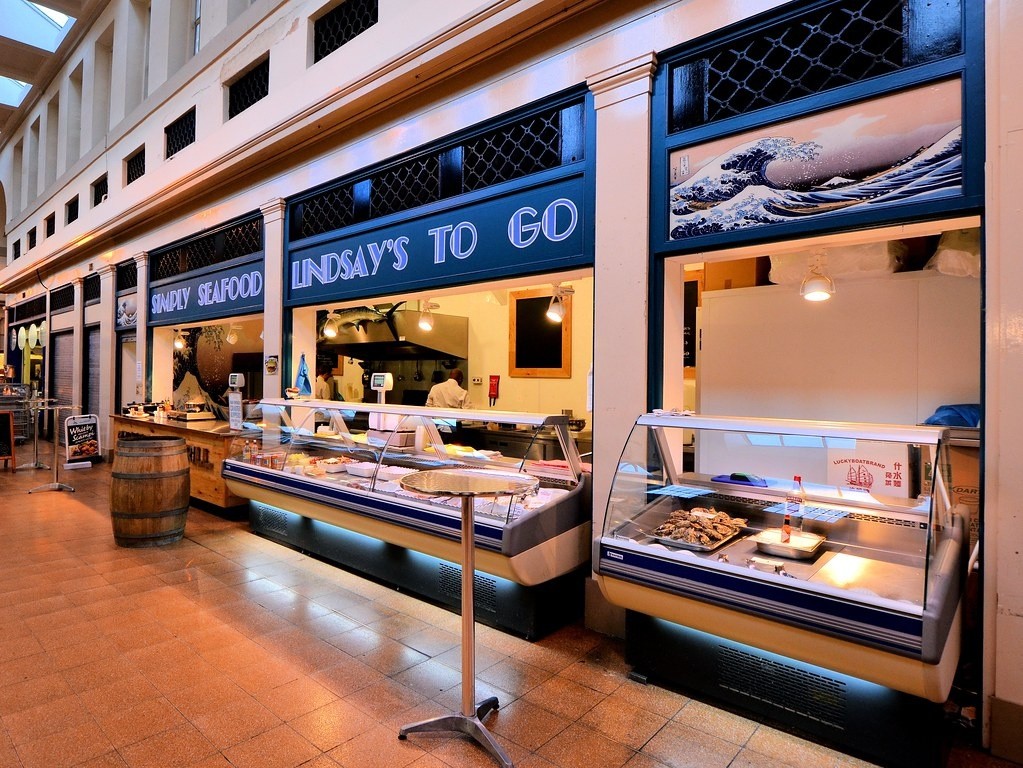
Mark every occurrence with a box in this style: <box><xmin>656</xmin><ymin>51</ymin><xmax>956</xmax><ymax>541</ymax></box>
<box><xmin>369</xmin><ymin>373</ymin><xmax>422</xmax><ymax>432</ymax></box>
<box><xmin>229</xmin><ymin>373</ymin><xmax>263</xmax><ymax>418</ymax></box>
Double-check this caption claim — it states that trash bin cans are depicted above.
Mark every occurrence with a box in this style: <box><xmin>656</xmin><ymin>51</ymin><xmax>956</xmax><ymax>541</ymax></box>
<box><xmin>110</xmin><ymin>435</ymin><xmax>191</xmax><ymax>548</ymax></box>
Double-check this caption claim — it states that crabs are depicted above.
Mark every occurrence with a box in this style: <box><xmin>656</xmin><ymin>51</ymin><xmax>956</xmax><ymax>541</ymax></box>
<box><xmin>655</xmin><ymin>506</ymin><xmax>749</xmax><ymax>545</ymax></box>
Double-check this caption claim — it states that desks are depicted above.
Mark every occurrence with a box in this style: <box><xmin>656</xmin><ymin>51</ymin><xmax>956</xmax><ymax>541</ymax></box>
<box><xmin>28</xmin><ymin>405</ymin><xmax>83</xmax><ymax>492</ymax></box>
<box><xmin>398</xmin><ymin>468</ymin><xmax>540</xmax><ymax>768</ymax></box>
<box><xmin>16</xmin><ymin>399</ymin><xmax>63</xmax><ymax>470</ymax></box>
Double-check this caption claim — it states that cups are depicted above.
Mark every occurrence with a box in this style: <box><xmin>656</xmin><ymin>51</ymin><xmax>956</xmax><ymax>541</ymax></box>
<box><xmin>284</xmin><ymin>465</ymin><xmax>313</xmax><ymax>476</ymax></box>
<box><xmin>154</xmin><ymin>411</ymin><xmax>167</xmax><ymax>418</ymax></box>
<box><xmin>138</xmin><ymin>406</ymin><xmax>143</xmax><ymax>413</ymax></box>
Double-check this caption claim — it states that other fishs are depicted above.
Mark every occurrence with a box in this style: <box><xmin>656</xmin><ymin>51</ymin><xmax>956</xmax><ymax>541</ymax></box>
<box><xmin>320</xmin><ymin>300</ymin><xmax>407</xmax><ymax>342</ymax></box>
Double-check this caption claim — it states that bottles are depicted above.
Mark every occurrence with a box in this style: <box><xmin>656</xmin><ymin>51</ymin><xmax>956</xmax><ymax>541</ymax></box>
<box><xmin>242</xmin><ymin>439</ymin><xmax>250</xmax><ymax>463</ymax></box>
<box><xmin>782</xmin><ymin>514</ymin><xmax>791</xmax><ymax>544</ymax></box>
<box><xmin>157</xmin><ymin>396</ymin><xmax>173</xmax><ymax>411</ymax></box>
<box><xmin>251</xmin><ymin>439</ymin><xmax>259</xmax><ymax>456</ymax></box>
<box><xmin>784</xmin><ymin>475</ymin><xmax>807</xmax><ymax>533</ymax></box>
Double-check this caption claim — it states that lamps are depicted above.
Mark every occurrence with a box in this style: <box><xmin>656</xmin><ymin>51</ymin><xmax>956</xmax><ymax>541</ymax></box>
<box><xmin>226</xmin><ymin>322</ymin><xmax>243</xmax><ymax>344</ymax></box>
<box><xmin>418</xmin><ymin>297</ymin><xmax>440</xmax><ymax>331</ymax></box>
<box><xmin>174</xmin><ymin>327</ymin><xmax>190</xmax><ymax>349</ymax></box>
<box><xmin>322</xmin><ymin>308</ymin><xmax>341</xmax><ymax>337</ymax></box>
<box><xmin>799</xmin><ymin>247</ymin><xmax>837</xmax><ymax>301</ymax></box>
<box><xmin>547</xmin><ymin>281</ymin><xmax>574</xmax><ymax>324</ymax></box>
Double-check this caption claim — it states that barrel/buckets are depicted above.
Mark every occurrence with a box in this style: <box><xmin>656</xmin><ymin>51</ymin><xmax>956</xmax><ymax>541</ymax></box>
<box><xmin>108</xmin><ymin>434</ymin><xmax>192</xmax><ymax>549</ymax></box>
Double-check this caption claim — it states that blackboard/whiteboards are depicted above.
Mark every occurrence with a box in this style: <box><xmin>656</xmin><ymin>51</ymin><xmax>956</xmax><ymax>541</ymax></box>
<box><xmin>0</xmin><ymin>410</ymin><xmax>15</xmax><ymax>459</ymax></box>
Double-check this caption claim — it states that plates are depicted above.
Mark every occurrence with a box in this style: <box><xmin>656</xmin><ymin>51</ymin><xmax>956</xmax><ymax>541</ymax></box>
<box><xmin>125</xmin><ymin>413</ymin><xmax>149</xmax><ymax>418</ymax></box>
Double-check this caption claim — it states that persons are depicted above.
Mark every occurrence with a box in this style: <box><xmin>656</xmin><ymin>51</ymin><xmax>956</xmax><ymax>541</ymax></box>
<box><xmin>314</xmin><ymin>365</ymin><xmax>332</xmax><ymax>434</ymax></box>
<box><xmin>424</xmin><ymin>367</ymin><xmax>471</xmax><ymax>447</ymax></box>
